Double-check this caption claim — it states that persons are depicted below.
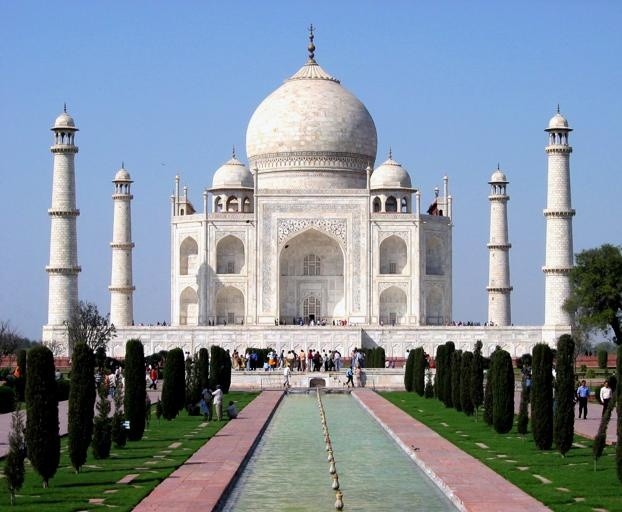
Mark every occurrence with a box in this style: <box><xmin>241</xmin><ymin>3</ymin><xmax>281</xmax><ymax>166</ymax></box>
<box><xmin>55</xmin><ymin>369</ymin><xmax>61</xmax><ymax>380</ymax></box>
<box><xmin>226</xmin><ymin>347</ymin><xmax>366</xmax><ymax>388</ymax></box>
<box><xmin>200</xmin><ymin>396</ymin><xmax>210</xmax><ymax>420</ymax></box>
<box><xmin>273</xmin><ymin>316</ymin><xmax>347</xmax><ymax>326</ymax></box>
<box><xmin>384</xmin><ymin>360</ymin><xmax>395</xmax><ymax>368</ymax></box>
<box><xmin>521</xmin><ymin>365</ymin><xmax>590</xmax><ymax>419</ymax></box>
<box><xmin>10</xmin><ymin>367</ymin><xmax>23</xmax><ymax>378</ymax></box>
<box><xmin>599</xmin><ymin>380</ymin><xmax>612</xmax><ymax>416</ymax></box>
<box><xmin>202</xmin><ymin>385</ymin><xmax>213</xmax><ymax>421</ymax></box>
<box><xmin>446</xmin><ymin>320</ymin><xmax>498</xmax><ymax>327</ymax></box>
<box><xmin>91</xmin><ymin>358</ymin><xmax>158</xmax><ymax>398</ymax></box>
<box><xmin>381</xmin><ymin>320</ymin><xmax>383</xmax><ymax>326</ymax></box>
<box><xmin>392</xmin><ymin>318</ymin><xmax>396</xmax><ymax>326</ymax></box>
<box><xmin>223</xmin><ymin>400</ymin><xmax>237</xmax><ymax>420</ymax></box>
<box><xmin>211</xmin><ymin>385</ymin><xmax>223</xmax><ymax>421</ymax></box>
